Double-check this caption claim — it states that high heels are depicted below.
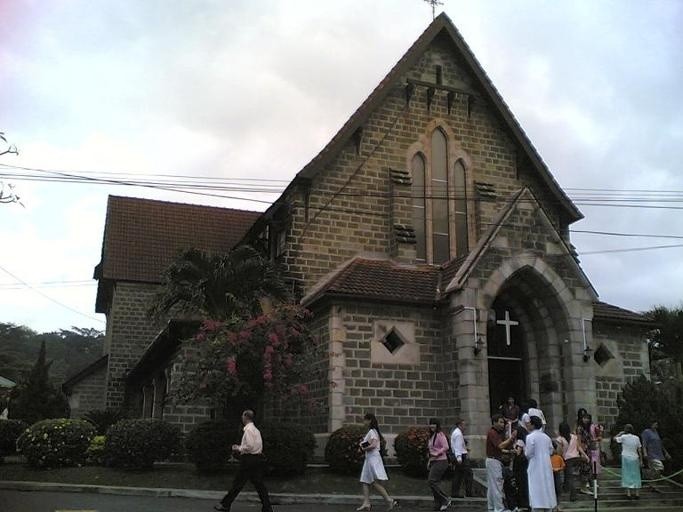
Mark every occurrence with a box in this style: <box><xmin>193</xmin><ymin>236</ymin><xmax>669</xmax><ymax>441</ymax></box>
<box><xmin>356</xmin><ymin>498</ymin><xmax>399</xmax><ymax>511</ymax></box>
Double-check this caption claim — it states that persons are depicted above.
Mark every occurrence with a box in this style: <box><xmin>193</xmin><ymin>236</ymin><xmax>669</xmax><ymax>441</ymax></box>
<box><xmin>356</xmin><ymin>414</ymin><xmax>398</xmax><ymax>512</ymax></box>
<box><xmin>486</xmin><ymin>397</ymin><xmax>602</xmax><ymax>512</ymax></box>
<box><xmin>613</xmin><ymin>419</ymin><xmax>671</xmax><ymax>500</ymax></box>
<box><xmin>215</xmin><ymin>410</ymin><xmax>273</xmax><ymax>512</ymax></box>
<box><xmin>427</xmin><ymin>418</ymin><xmax>473</xmax><ymax>512</ymax></box>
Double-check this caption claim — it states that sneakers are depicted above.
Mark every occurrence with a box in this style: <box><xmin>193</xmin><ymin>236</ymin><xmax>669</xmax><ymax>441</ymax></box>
<box><xmin>440</xmin><ymin>497</ymin><xmax>454</xmax><ymax>510</ymax></box>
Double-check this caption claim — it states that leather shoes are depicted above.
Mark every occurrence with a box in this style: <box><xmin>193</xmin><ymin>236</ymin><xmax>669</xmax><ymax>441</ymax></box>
<box><xmin>213</xmin><ymin>504</ymin><xmax>274</xmax><ymax>512</ymax></box>
